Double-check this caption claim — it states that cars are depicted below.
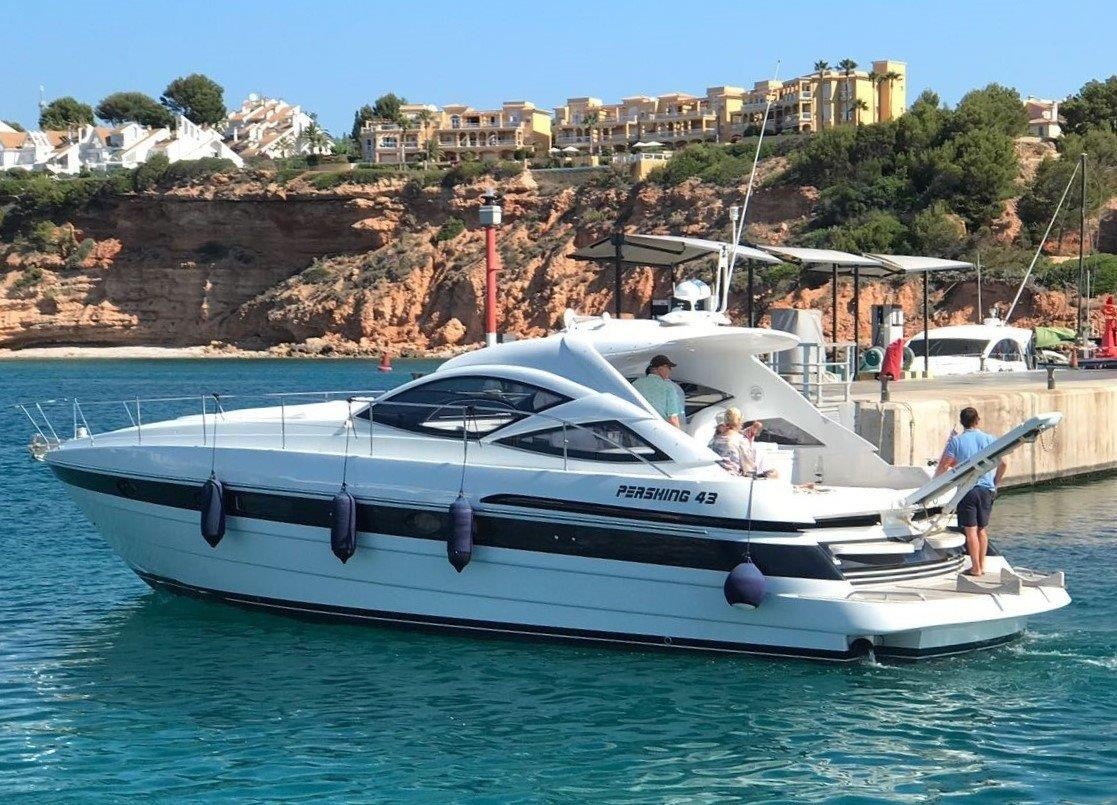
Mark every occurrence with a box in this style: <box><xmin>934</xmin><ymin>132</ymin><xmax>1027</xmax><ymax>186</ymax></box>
<box><xmin>901</xmin><ymin>324</ymin><xmax>1042</xmax><ymax>376</ymax></box>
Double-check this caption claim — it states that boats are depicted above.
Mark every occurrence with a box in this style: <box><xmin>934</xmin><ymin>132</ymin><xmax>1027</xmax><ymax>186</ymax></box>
<box><xmin>43</xmin><ymin>310</ymin><xmax>1074</xmax><ymax>667</ymax></box>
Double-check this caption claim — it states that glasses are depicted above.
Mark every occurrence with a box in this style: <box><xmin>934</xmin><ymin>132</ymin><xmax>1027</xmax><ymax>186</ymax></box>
<box><xmin>663</xmin><ymin>364</ymin><xmax>671</xmax><ymax>369</ymax></box>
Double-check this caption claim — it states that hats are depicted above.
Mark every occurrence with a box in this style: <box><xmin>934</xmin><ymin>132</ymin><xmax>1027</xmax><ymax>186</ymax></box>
<box><xmin>650</xmin><ymin>355</ymin><xmax>677</xmax><ymax>367</ymax></box>
<box><xmin>714</xmin><ymin>412</ymin><xmax>728</xmax><ymax>427</ymax></box>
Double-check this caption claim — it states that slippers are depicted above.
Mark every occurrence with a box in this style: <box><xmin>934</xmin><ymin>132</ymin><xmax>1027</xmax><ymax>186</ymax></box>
<box><xmin>962</xmin><ymin>567</ymin><xmax>985</xmax><ymax>576</ymax></box>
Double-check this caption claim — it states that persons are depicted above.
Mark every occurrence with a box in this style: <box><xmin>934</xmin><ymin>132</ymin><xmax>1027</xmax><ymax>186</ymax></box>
<box><xmin>935</xmin><ymin>407</ymin><xmax>1007</xmax><ymax>576</ymax></box>
<box><xmin>632</xmin><ymin>355</ymin><xmax>685</xmax><ymax>429</ymax></box>
<box><xmin>708</xmin><ymin>410</ymin><xmax>762</xmax><ymax>476</ymax></box>
